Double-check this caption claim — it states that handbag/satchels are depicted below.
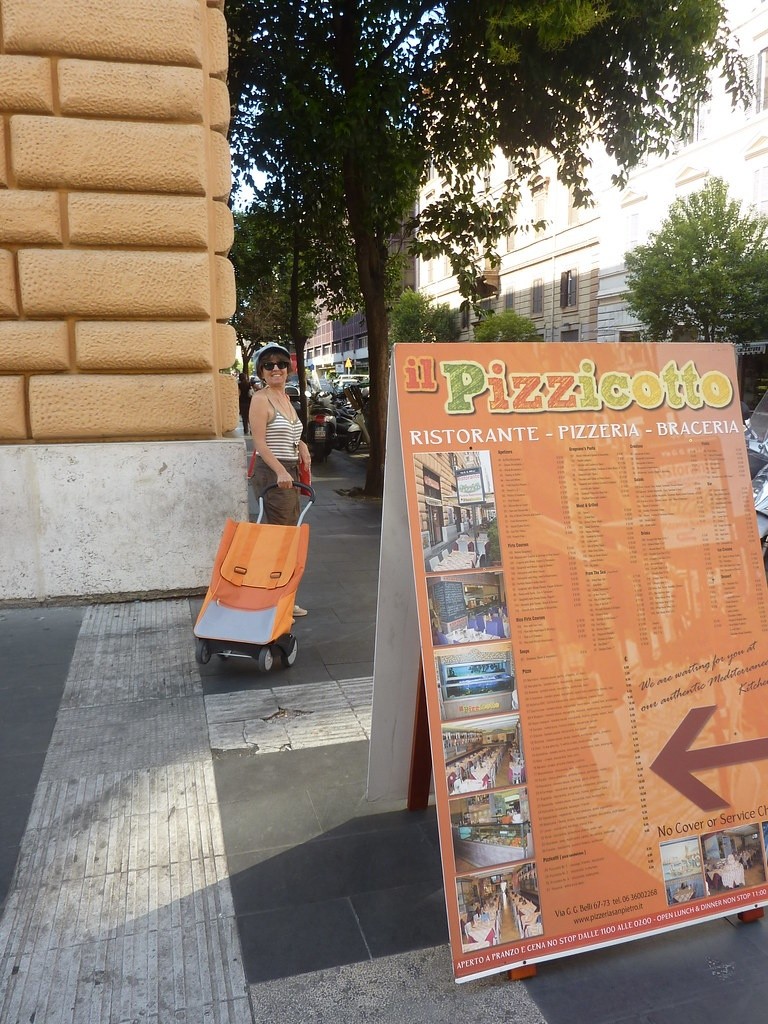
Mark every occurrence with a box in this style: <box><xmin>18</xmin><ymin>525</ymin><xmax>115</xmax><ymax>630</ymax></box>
<box><xmin>248</xmin><ymin>382</ymin><xmax>254</xmax><ymax>399</ymax></box>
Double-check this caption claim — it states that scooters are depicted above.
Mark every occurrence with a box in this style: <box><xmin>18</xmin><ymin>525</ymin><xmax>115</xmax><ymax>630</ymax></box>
<box><xmin>301</xmin><ymin>382</ymin><xmax>370</xmax><ymax>464</ymax></box>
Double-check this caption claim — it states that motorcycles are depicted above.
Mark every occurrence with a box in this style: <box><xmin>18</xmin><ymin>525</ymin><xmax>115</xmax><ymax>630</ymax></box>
<box><xmin>741</xmin><ymin>389</ymin><xmax>768</xmax><ymax>571</ymax></box>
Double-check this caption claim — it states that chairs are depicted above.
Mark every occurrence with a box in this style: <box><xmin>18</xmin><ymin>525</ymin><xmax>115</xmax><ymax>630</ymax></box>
<box><xmin>479</xmin><ymin>554</ymin><xmax>486</xmax><ymax>568</ymax></box>
<box><xmin>428</xmin><ymin>556</ymin><xmax>440</xmax><ymax>571</ymax></box>
<box><xmin>436</xmin><ymin>606</ymin><xmax>507</xmax><ymax>645</ymax></box>
<box><xmin>441</xmin><ymin>549</ymin><xmax>449</xmax><ymax>558</ymax></box>
<box><xmin>468</xmin><ymin>543</ymin><xmax>474</xmax><ymax>552</ymax></box>
<box><xmin>484</xmin><ymin>542</ymin><xmax>491</xmax><ymax>557</ymax></box>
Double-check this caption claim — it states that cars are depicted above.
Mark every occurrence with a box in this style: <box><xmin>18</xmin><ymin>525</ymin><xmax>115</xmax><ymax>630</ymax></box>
<box><xmin>308</xmin><ymin>380</ymin><xmax>332</xmax><ymax>395</ymax></box>
<box><xmin>337</xmin><ymin>375</ymin><xmax>351</xmax><ymax>379</ymax></box>
<box><xmin>334</xmin><ymin>379</ymin><xmax>359</xmax><ymax>394</ymax></box>
<box><xmin>351</xmin><ymin>376</ymin><xmax>370</xmax><ymax>382</ymax></box>
<box><xmin>441</xmin><ymin>661</ymin><xmax>514</xmax><ymax>697</ymax></box>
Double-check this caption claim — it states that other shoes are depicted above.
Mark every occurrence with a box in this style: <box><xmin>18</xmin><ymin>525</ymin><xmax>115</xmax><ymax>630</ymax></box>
<box><xmin>292</xmin><ymin>605</ymin><xmax>308</xmax><ymax>617</ymax></box>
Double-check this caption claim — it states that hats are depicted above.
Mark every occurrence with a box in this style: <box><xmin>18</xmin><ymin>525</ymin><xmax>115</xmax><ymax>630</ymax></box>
<box><xmin>252</xmin><ymin>341</ymin><xmax>290</xmax><ymax>380</ymax></box>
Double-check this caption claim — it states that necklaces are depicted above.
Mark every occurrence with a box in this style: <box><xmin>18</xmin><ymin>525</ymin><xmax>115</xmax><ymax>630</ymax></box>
<box><xmin>276</xmin><ymin>396</ymin><xmax>298</xmax><ymax>456</ymax></box>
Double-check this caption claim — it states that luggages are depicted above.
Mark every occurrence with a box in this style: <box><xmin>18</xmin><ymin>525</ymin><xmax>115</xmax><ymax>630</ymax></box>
<box><xmin>193</xmin><ymin>482</ymin><xmax>317</xmax><ymax>672</ymax></box>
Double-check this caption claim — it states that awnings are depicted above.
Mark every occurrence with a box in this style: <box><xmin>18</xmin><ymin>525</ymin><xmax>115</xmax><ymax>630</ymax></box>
<box><xmin>733</xmin><ymin>340</ymin><xmax>768</xmax><ymax>356</ymax></box>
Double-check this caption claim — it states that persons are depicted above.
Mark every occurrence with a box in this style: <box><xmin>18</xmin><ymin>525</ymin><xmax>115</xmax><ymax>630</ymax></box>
<box><xmin>248</xmin><ymin>343</ymin><xmax>313</xmax><ymax>623</ymax></box>
<box><xmin>237</xmin><ymin>372</ymin><xmax>252</xmax><ymax>435</ymax></box>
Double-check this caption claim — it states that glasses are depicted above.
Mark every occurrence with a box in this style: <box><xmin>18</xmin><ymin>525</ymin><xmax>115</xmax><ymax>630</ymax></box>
<box><xmin>263</xmin><ymin>361</ymin><xmax>289</xmax><ymax>371</ymax></box>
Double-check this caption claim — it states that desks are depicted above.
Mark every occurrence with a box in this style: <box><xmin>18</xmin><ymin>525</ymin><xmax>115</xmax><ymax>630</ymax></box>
<box><xmin>462</xmin><ymin>892</ymin><xmax>544</xmax><ymax>947</ymax></box>
<box><xmin>433</xmin><ymin>549</ymin><xmax>477</xmax><ymax>571</ymax></box>
<box><xmin>447</xmin><ymin>747</ymin><xmax>525</xmax><ymax>795</ymax></box>
<box><xmin>673</xmin><ymin>886</ymin><xmax>695</xmax><ymax>904</ymax></box>
<box><xmin>445</xmin><ymin>630</ymin><xmax>500</xmax><ymax>643</ymax></box>
<box><xmin>708</xmin><ymin>848</ymin><xmax>757</xmax><ymax>892</ymax></box>
